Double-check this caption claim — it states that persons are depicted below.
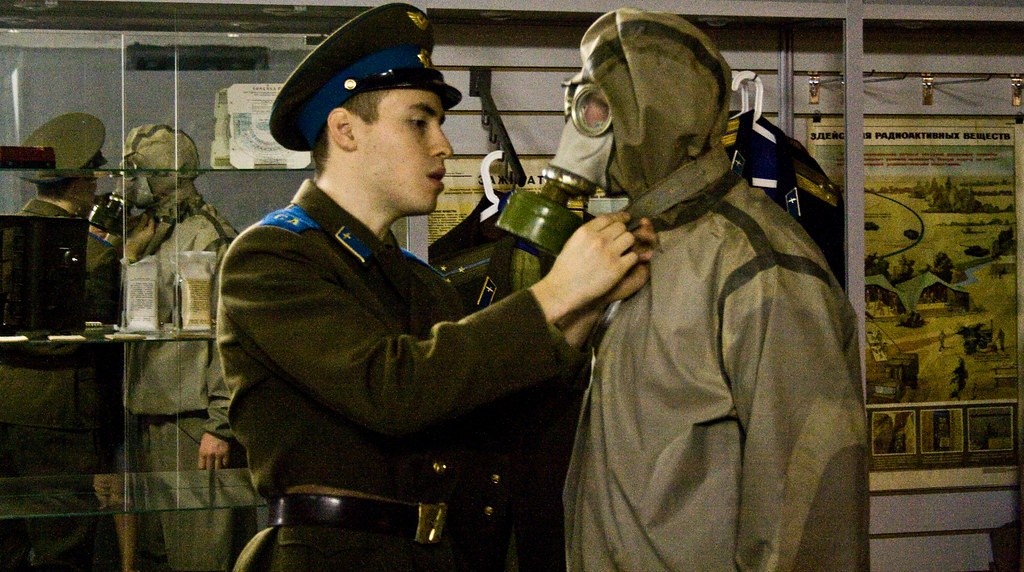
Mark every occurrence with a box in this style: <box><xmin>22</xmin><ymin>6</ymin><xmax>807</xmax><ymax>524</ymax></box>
<box><xmin>88</xmin><ymin>124</ymin><xmax>240</xmax><ymax>572</ymax></box>
<box><xmin>0</xmin><ymin>112</ymin><xmax>154</xmax><ymax>572</ymax></box>
<box><xmin>216</xmin><ymin>2</ymin><xmax>658</xmax><ymax>572</ymax></box>
<box><xmin>494</xmin><ymin>8</ymin><xmax>868</xmax><ymax>572</ymax></box>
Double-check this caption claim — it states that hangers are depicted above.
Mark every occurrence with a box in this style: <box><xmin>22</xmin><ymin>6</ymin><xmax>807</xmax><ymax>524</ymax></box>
<box><xmin>729</xmin><ymin>70</ymin><xmax>776</xmax><ymax>144</ymax></box>
<box><xmin>481</xmin><ymin>150</ymin><xmax>504</xmax><ymax>222</ymax></box>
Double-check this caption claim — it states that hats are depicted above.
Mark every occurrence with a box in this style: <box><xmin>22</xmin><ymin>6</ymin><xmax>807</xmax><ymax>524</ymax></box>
<box><xmin>15</xmin><ymin>113</ymin><xmax>108</xmax><ymax>183</ymax></box>
<box><xmin>269</xmin><ymin>4</ymin><xmax>462</xmax><ymax>152</ymax></box>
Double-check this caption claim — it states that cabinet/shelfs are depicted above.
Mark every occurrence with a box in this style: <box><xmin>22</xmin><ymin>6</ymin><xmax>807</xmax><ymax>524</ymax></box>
<box><xmin>0</xmin><ymin>168</ymin><xmax>317</xmax><ymax>518</ymax></box>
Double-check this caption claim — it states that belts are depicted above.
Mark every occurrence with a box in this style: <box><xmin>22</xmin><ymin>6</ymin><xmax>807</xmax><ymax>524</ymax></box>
<box><xmin>269</xmin><ymin>492</ymin><xmax>462</xmax><ymax>544</ymax></box>
